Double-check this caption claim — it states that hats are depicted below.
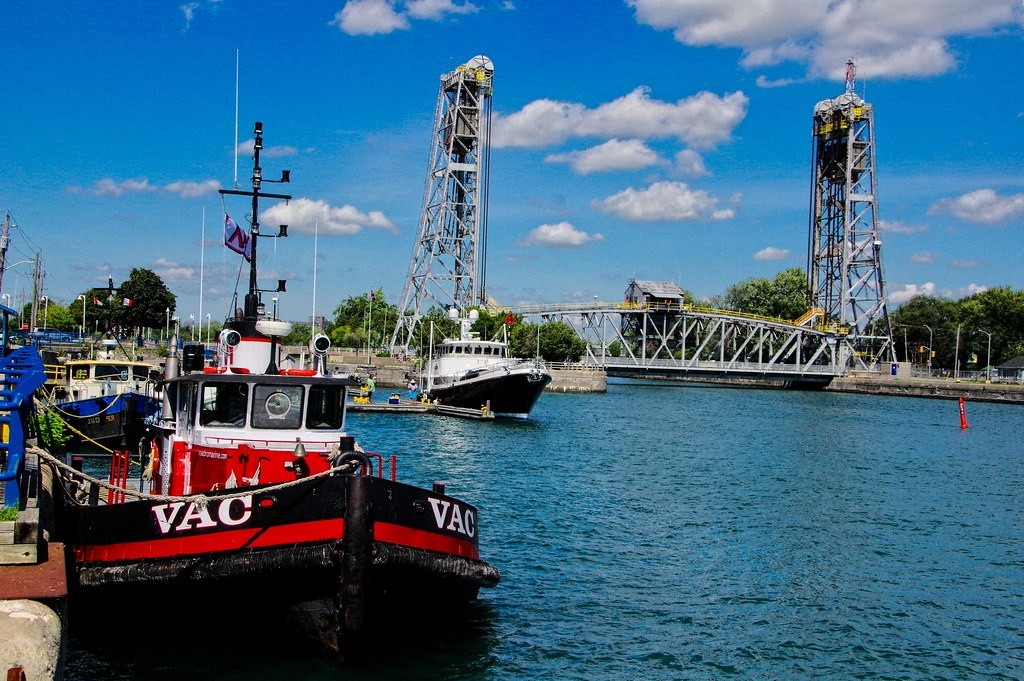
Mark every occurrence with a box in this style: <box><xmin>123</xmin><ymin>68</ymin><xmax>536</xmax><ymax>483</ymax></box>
<box><xmin>411</xmin><ymin>379</ymin><xmax>416</xmax><ymax>384</ymax></box>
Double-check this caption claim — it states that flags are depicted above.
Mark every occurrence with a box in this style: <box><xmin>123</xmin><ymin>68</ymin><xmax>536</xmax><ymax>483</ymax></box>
<box><xmin>224</xmin><ymin>214</ymin><xmax>255</xmax><ymax>262</ymax></box>
<box><xmin>94</xmin><ymin>297</ymin><xmax>103</xmax><ymax>306</ymax></box>
<box><xmin>123</xmin><ymin>297</ymin><xmax>133</xmax><ymax>306</ymax></box>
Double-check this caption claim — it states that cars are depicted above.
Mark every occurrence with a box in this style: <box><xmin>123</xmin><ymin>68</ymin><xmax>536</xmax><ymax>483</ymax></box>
<box><xmin>33</xmin><ymin>327</ymin><xmax>70</xmax><ymax>342</ymax></box>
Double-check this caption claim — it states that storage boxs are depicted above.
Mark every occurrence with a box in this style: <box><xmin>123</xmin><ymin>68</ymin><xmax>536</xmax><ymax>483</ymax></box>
<box><xmin>389</xmin><ymin>397</ymin><xmax>399</xmax><ymax>404</ymax></box>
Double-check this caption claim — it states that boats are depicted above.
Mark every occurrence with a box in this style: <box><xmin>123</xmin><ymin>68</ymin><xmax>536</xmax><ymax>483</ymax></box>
<box><xmin>47</xmin><ymin>275</ymin><xmax>166</xmax><ymax>453</ymax></box>
<box><xmin>57</xmin><ymin>47</ymin><xmax>500</xmax><ymax>680</ymax></box>
<box><xmin>421</xmin><ymin>306</ymin><xmax>552</xmax><ymax>420</ymax></box>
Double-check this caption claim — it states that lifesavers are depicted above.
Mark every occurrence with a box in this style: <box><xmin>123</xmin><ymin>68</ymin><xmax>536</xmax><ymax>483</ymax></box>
<box><xmin>149</xmin><ymin>441</ymin><xmax>160</xmax><ymax>480</ymax></box>
<box><xmin>333</xmin><ymin>450</ymin><xmax>373</xmax><ymax>476</ymax></box>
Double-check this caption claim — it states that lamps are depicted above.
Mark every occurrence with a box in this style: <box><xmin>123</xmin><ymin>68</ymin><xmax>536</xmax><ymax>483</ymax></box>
<box><xmin>311</xmin><ymin>334</ymin><xmax>331</xmax><ymax>377</ymax></box>
<box><xmin>494</xmin><ymin>333</ymin><xmax>500</xmax><ymax>342</ymax></box>
<box><xmin>218</xmin><ymin>330</ymin><xmax>241</xmax><ymax>375</ymax></box>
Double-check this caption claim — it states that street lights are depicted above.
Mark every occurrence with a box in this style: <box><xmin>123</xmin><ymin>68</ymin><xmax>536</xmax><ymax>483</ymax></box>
<box><xmin>973</xmin><ymin>330</ymin><xmax>991</xmax><ymax>380</ymax></box>
<box><xmin>172</xmin><ymin>317</ymin><xmax>179</xmax><ymax>340</ymax></box>
<box><xmin>206</xmin><ymin>314</ymin><xmax>210</xmax><ymax>349</ymax></box>
<box><xmin>166</xmin><ymin>308</ymin><xmax>170</xmax><ymax>338</ymax></box>
<box><xmin>190</xmin><ymin>314</ymin><xmax>194</xmax><ymax>341</ymax></box>
<box><xmin>923</xmin><ymin>325</ymin><xmax>932</xmax><ymax>372</ymax></box>
<box><xmin>2</xmin><ymin>294</ymin><xmax>10</xmax><ymax>320</ymax></box>
<box><xmin>41</xmin><ymin>295</ymin><xmax>48</xmax><ymax>330</ymax></box>
<box><xmin>78</xmin><ymin>295</ymin><xmax>86</xmax><ymax>333</ymax></box>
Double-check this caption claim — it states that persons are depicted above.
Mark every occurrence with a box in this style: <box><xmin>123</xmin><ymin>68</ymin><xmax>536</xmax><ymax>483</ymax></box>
<box><xmin>365</xmin><ymin>375</ymin><xmax>375</xmax><ymax>403</ymax></box>
<box><xmin>408</xmin><ymin>379</ymin><xmax>418</xmax><ymax>405</ymax></box>
